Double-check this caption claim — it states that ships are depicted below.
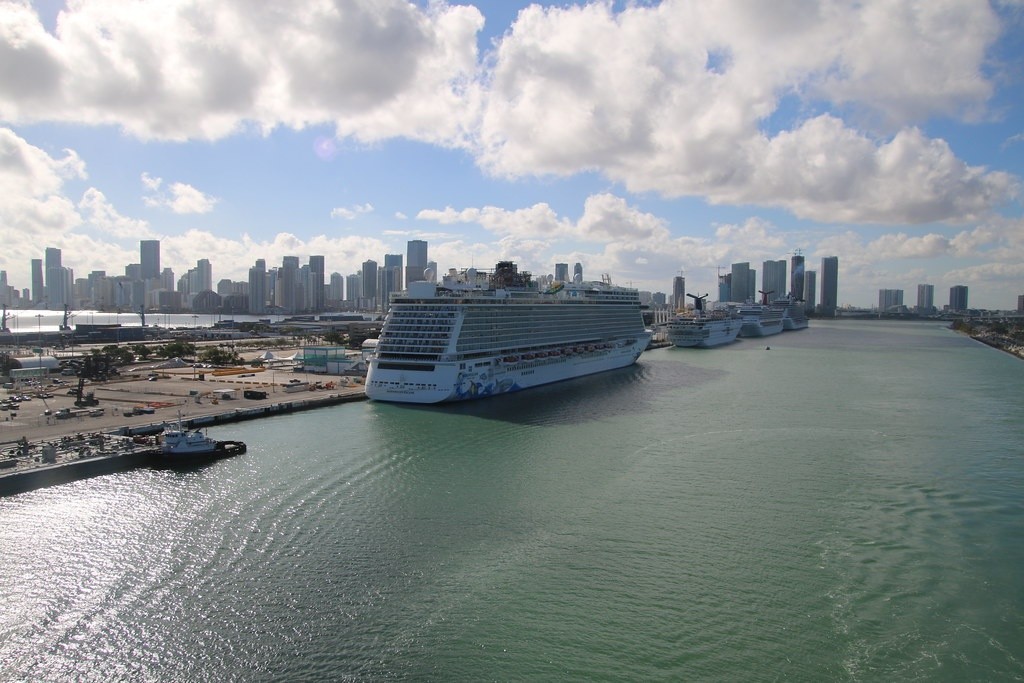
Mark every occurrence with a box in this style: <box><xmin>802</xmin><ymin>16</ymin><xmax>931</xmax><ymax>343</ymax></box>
<box><xmin>364</xmin><ymin>263</ymin><xmax>653</xmax><ymax>404</ymax></box>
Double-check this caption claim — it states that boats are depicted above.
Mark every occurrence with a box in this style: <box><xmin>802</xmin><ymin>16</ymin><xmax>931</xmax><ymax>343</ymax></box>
<box><xmin>666</xmin><ymin>293</ymin><xmax>742</xmax><ymax>347</ymax></box>
<box><xmin>737</xmin><ymin>291</ymin><xmax>809</xmax><ymax>336</ymax></box>
<box><xmin>142</xmin><ymin>408</ymin><xmax>246</xmax><ymax>461</ymax></box>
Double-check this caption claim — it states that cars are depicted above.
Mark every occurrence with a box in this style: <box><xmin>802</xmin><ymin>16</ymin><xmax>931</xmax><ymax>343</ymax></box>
<box><xmin>149</xmin><ymin>377</ymin><xmax>156</xmax><ymax>381</ymax></box>
<box><xmin>24</xmin><ymin>377</ymin><xmax>59</xmax><ymax>385</ymax></box>
<box><xmin>89</xmin><ymin>408</ymin><xmax>155</xmax><ymax>417</ymax></box>
<box><xmin>45</xmin><ymin>409</ymin><xmax>52</xmax><ymax>415</ymax></box>
<box><xmin>193</xmin><ymin>363</ymin><xmax>202</xmax><ymax>368</ymax></box>
<box><xmin>0</xmin><ymin>393</ymin><xmax>54</xmax><ymax>411</ymax></box>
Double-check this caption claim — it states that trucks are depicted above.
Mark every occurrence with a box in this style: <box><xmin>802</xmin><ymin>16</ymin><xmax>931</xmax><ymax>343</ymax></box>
<box><xmin>62</xmin><ymin>369</ymin><xmax>73</xmax><ymax>375</ymax></box>
<box><xmin>55</xmin><ymin>408</ymin><xmax>71</xmax><ymax>419</ymax></box>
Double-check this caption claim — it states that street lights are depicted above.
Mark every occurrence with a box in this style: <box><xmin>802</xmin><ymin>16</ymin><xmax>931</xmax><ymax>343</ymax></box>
<box><xmin>192</xmin><ymin>313</ymin><xmax>200</xmax><ymax>382</ymax></box>
<box><xmin>36</xmin><ymin>313</ymin><xmax>43</xmax><ymax>391</ymax></box>
